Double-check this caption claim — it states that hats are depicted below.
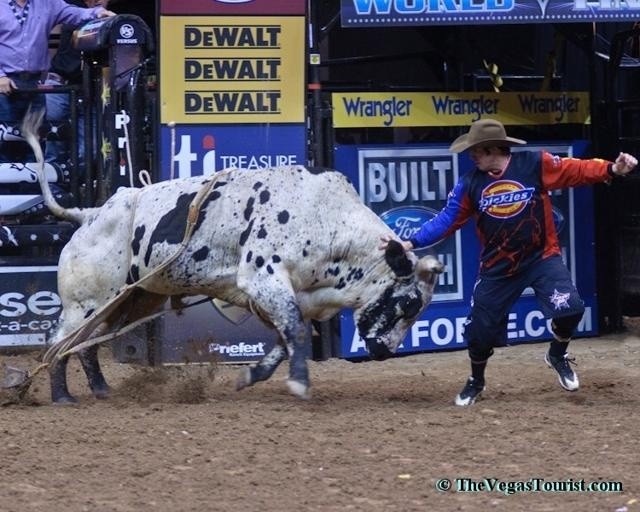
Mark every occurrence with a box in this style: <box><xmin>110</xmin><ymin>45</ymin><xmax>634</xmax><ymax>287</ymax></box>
<box><xmin>449</xmin><ymin>119</ymin><xmax>527</xmax><ymax>153</ymax></box>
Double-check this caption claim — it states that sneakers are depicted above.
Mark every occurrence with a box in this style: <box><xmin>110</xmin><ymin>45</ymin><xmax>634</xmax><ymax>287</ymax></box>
<box><xmin>544</xmin><ymin>352</ymin><xmax>579</xmax><ymax>391</ymax></box>
<box><xmin>455</xmin><ymin>376</ymin><xmax>484</xmax><ymax>406</ymax></box>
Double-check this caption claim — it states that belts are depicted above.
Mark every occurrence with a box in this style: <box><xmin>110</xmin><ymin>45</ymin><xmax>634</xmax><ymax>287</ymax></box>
<box><xmin>10</xmin><ymin>72</ymin><xmax>46</xmax><ymax>81</ymax></box>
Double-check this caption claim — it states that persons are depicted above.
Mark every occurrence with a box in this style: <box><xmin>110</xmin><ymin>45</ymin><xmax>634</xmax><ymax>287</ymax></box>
<box><xmin>43</xmin><ymin>1</ymin><xmax>112</xmax><ymax>187</ymax></box>
<box><xmin>0</xmin><ymin>1</ymin><xmax>118</xmax><ymax>126</ymax></box>
<box><xmin>376</xmin><ymin>118</ymin><xmax>639</xmax><ymax>408</ymax></box>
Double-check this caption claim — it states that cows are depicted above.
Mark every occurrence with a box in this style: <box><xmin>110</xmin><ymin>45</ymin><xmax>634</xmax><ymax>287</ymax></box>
<box><xmin>20</xmin><ymin>107</ymin><xmax>445</xmax><ymax>404</ymax></box>
